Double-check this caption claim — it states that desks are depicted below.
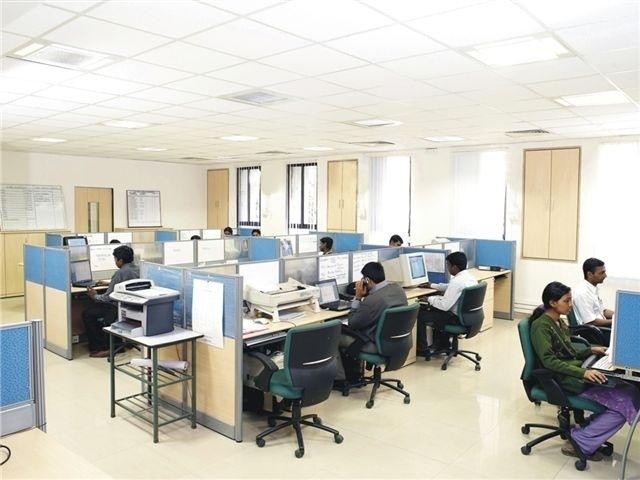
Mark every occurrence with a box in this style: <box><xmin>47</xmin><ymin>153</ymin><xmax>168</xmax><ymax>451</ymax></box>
<box><xmin>590</xmin><ymin>288</ymin><xmax>640</xmax><ymax>480</ymax></box>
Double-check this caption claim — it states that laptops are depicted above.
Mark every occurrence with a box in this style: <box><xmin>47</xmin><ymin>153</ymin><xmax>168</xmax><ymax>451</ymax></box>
<box><xmin>311</xmin><ymin>279</ymin><xmax>354</xmax><ymax>311</ymax></box>
<box><xmin>66</xmin><ymin>259</ymin><xmax>101</xmax><ymax>288</ymax></box>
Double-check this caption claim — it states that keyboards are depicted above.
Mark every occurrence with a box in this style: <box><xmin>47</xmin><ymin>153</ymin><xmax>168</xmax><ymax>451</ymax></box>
<box><xmin>591</xmin><ymin>350</ymin><xmax>617</xmax><ymax>372</ymax></box>
<box><xmin>420</xmin><ymin>293</ymin><xmax>445</xmax><ymax>301</ymax></box>
<box><xmin>244</xmin><ymin>331</ymin><xmax>288</xmax><ymax>347</ymax></box>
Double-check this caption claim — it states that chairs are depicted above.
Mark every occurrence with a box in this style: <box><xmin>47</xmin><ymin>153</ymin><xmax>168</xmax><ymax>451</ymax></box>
<box><xmin>517</xmin><ymin>316</ymin><xmax>615</xmax><ymax>470</ymax></box>
<box><xmin>567</xmin><ymin>306</ymin><xmax>611</xmax><ymax>343</ymax></box>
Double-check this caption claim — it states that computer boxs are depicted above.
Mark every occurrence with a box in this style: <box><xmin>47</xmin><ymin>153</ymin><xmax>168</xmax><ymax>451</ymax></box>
<box><xmin>245</xmin><ymin>351</ymin><xmax>286</xmax><ymax>415</ymax></box>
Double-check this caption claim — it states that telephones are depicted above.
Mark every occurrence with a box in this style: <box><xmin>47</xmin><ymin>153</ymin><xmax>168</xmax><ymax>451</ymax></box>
<box><xmin>363</xmin><ymin>280</ymin><xmax>369</xmax><ymax>297</ymax></box>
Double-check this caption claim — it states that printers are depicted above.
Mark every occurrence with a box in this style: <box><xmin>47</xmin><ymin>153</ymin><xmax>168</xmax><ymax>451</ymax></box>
<box><xmin>110</xmin><ymin>278</ymin><xmax>182</xmax><ymax>337</ymax></box>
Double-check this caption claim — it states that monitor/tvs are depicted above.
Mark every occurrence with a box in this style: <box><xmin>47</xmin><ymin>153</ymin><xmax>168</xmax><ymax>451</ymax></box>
<box><xmin>63</xmin><ymin>234</ymin><xmax>90</xmax><ymax>247</ymax></box>
<box><xmin>381</xmin><ymin>252</ymin><xmax>432</xmax><ymax>290</ymax></box>
<box><xmin>458</xmin><ymin>239</ymin><xmax>477</xmax><ymax>269</ymax></box>
<box><xmin>279</xmin><ymin>239</ymin><xmax>296</xmax><ymax>259</ymax></box>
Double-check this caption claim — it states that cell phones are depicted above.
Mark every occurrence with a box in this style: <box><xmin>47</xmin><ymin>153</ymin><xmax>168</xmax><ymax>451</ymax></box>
<box><xmin>361</xmin><ymin>277</ymin><xmax>369</xmax><ymax>286</ymax></box>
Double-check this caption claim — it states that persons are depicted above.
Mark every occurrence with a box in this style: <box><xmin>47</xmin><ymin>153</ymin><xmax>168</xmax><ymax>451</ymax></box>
<box><xmin>416</xmin><ymin>251</ymin><xmax>480</xmax><ymax>356</ymax></box>
<box><xmin>571</xmin><ymin>257</ymin><xmax>615</xmax><ymax>346</ymax></box>
<box><xmin>528</xmin><ymin>280</ymin><xmax>639</xmax><ymax>462</ymax></box>
<box><xmin>251</xmin><ymin>229</ymin><xmax>260</xmax><ymax>236</ymax></box>
<box><xmin>389</xmin><ymin>234</ymin><xmax>404</xmax><ymax>248</ymax></box>
<box><xmin>224</xmin><ymin>227</ymin><xmax>232</xmax><ymax>236</ymax></box>
<box><xmin>332</xmin><ymin>261</ymin><xmax>408</xmax><ymax>391</ymax></box>
<box><xmin>319</xmin><ymin>236</ymin><xmax>334</xmax><ymax>256</ymax></box>
<box><xmin>81</xmin><ymin>245</ymin><xmax>141</xmax><ymax>358</ymax></box>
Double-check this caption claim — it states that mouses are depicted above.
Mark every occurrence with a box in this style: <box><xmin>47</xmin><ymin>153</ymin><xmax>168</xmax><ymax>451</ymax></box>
<box><xmin>596</xmin><ymin>377</ymin><xmax>608</xmax><ymax>384</ymax></box>
<box><xmin>418</xmin><ymin>279</ymin><xmax>431</xmax><ymax>290</ymax></box>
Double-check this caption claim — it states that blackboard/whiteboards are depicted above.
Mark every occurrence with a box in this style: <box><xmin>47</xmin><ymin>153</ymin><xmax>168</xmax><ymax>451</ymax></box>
<box><xmin>126</xmin><ymin>190</ymin><xmax>162</xmax><ymax>228</ymax></box>
<box><xmin>0</xmin><ymin>184</ymin><xmax>72</xmax><ymax>233</ymax></box>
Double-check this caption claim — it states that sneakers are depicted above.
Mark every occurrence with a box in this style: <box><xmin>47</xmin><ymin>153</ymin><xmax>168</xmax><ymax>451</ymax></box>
<box><xmin>417</xmin><ymin>349</ymin><xmax>431</xmax><ymax>357</ymax></box>
<box><xmin>430</xmin><ymin>343</ymin><xmax>451</xmax><ymax>350</ymax></box>
<box><xmin>90</xmin><ymin>350</ymin><xmax>111</xmax><ymax>357</ymax></box>
<box><xmin>561</xmin><ymin>442</ymin><xmax>604</xmax><ymax>461</ymax></box>
